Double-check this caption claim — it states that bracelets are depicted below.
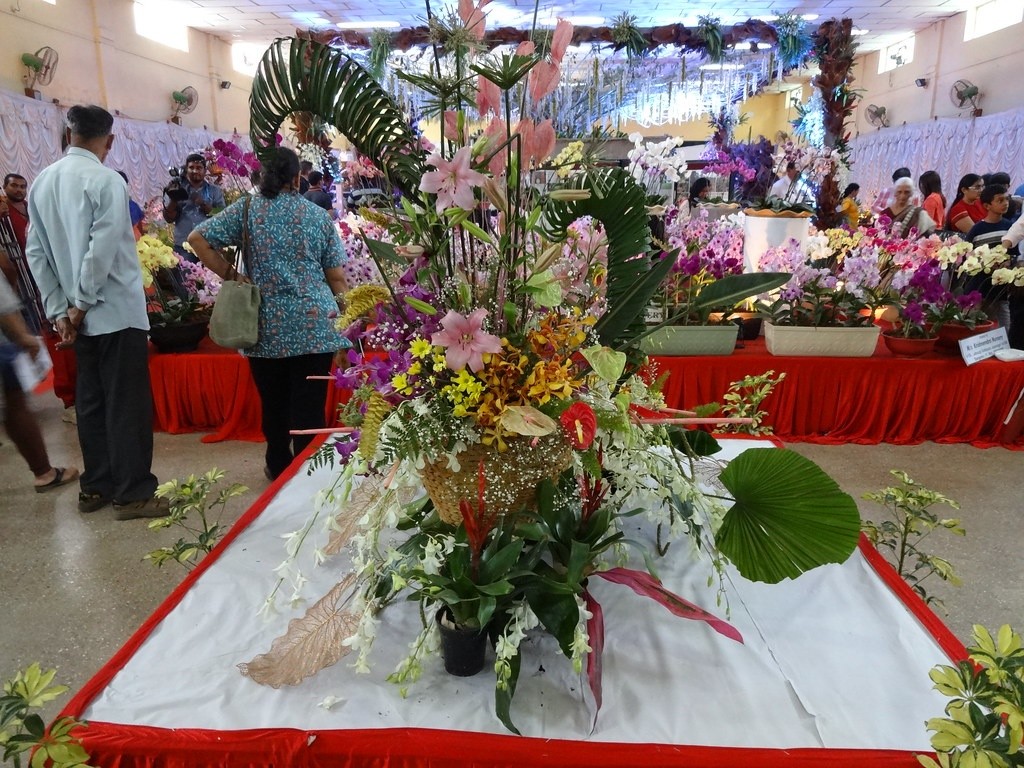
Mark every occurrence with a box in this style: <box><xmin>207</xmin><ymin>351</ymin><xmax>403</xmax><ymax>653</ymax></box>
<box><xmin>201</xmin><ymin>201</ymin><xmax>206</xmax><ymax>207</ymax></box>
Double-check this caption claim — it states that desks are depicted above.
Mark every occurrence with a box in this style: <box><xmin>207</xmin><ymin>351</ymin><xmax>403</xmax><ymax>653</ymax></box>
<box><xmin>29</xmin><ymin>427</ymin><xmax>1022</xmax><ymax>768</ymax></box>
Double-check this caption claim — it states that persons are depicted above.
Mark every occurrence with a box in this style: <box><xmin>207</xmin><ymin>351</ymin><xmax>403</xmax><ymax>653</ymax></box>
<box><xmin>0</xmin><ymin>171</ymin><xmax>80</xmax><ymax>492</ymax></box>
<box><xmin>163</xmin><ymin>153</ymin><xmax>226</xmax><ymax>301</ymax></box>
<box><xmin>299</xmin><ymin>161</ymin><xmax>333</xmax><ymax>217</ymax></box>
<box><xmin>188</xmin><ymin>146</ymin><xmax>350</xmax><ymax>482</ymax></box>
<box><xmin>870</xmin><ymin>168</ymin><xmax>1024</xmax><ymax>351</ymax></box>
<box><xmin>25</xmin><ymin>105</ymin><xmax>171</xmax><ymax>520</ymax></box>
<box><xmin>841</xmin><ymin>183</ymin><xmax>860</xmax><ymax>230</ymax></box>
<box><xmin>765</xmin><ymin>161</ymin><xmax>816</xmax><ymax>208</ymax></box>
<box><xmin>746</xmin><ymin>160</ymin><xmax>780</xmax><ymax>197</ymax></box>
<box><xmin>679</xmin><ymin>178</ymin><xmax>712</xmax><ymax>215</ymax></box>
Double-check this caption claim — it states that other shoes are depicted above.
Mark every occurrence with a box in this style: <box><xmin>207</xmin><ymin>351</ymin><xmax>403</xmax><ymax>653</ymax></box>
<box><xmin>79</xmin><ymin>491</ymin><xmax>111</xmax><ymax>512</ymax></box>
<box><xmin>113</xmin><ymin>495</ymin><xmax>172</xmax><ymax>520</ymax></box>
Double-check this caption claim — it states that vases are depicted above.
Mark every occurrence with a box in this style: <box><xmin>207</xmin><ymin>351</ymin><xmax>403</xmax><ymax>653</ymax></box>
<box><xmin>623</xmin><ymin>320</ymin><xmax>739</xmax><ymax>357</ymax></box>
<box><xmin>763</xmin><ymin>319</ymin><xmax>883</xmax><ymax>358</ymax></box>
<box><xmin>434</xmin><ymin>605</ymin><xmax>489</xmax><ymax>677</ymax></box>
<box><xmin>881</xmin><ymin>329</ymin><xmax>941</xmax><ymax>356</ymax></box>
<box><xmin>148</xmin><ymin>315</ymin><xmax>211</xmax><ymax>352</ymax></box>
<box><xmin>732</xmin><ymin>315</ymin><xmax>762</xmax><ymax>341</ymax></box>
<box><xmin>925</xmin><ymin>316</ymin><xmax>995</xmax><ymax>349</ymax></box>
<box><xmin>416</xmin><ymin>423</ymin><xmax>574</xmax><ymax>528</ymax></box>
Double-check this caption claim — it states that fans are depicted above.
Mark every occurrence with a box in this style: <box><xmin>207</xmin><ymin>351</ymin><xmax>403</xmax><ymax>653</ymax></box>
<box><xmin>949</xmin><ymin>78</ymin><xmax>984</xmax><ymax>116</ymax></box>
<box><xmin>171</xmin><ymin>86</ymin><xmax>199</xmax><ymax>124</ymax></box>
<box><xmin>20</xmin><ymin>46</ymin><xmax>59</xmax><ymax>91</ymax></box>
<box><xmin>864</xmin><ymin>104</ymin><xmax>890</xmax><ymax>129</ymax></box>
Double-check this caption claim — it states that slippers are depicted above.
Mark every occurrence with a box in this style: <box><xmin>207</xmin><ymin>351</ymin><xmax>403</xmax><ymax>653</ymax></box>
<box><xmin>33</xmin><ymin>467</ymin><xmax>80</xmax><ymax>494</ymax></box>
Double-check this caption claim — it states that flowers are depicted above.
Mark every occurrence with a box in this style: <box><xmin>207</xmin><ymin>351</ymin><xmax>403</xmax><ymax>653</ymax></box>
<box><xmin>0</xmin><ymin>0</ymin><xmax>1024</xmax><ymax>768</ymax></box>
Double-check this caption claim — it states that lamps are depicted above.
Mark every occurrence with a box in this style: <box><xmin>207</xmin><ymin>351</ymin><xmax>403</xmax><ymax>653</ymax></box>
<box><xmin>914</xmin><ymin>74</ymin><xmax>930</xmax><ymax>87</ymax></box>
<box><xmin>220</xmin><ymin>80</ymin><xmax>231</xmax><ymax>90</ymax></box>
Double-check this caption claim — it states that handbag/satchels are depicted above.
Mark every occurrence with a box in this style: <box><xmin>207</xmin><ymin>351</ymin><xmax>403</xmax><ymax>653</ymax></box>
<box><xmin>209</xmin><ymin>280</ymin><xmax>260</xmax><ymax>348</ymax></box>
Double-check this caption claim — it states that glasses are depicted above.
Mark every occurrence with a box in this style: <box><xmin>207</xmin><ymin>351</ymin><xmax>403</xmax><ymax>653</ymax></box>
<box><xmin>967</xmin><ymin>186</ymin><xmax>985</xmax><ymax>190</ymax></box>
